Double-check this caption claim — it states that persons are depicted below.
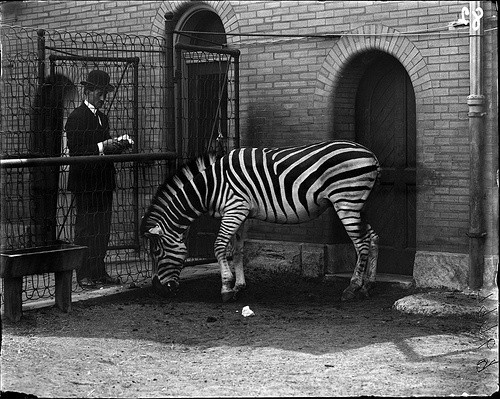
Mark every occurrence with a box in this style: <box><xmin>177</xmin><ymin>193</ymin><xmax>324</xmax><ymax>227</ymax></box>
<box><xmin>65</xmin><ymin>70</ymin><xmax>134</xmax><ymax>287</ymax></box>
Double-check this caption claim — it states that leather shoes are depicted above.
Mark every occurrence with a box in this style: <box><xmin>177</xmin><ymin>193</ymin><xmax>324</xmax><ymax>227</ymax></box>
<box><xmin>101</xmin><ymin>273</ymin><xmax>120</xmax><ymax>284</ymax></box>
<box><xmin>77</xmin><ymin>277</ymin><xmax>98</xmax><ymax>289</ymax></box>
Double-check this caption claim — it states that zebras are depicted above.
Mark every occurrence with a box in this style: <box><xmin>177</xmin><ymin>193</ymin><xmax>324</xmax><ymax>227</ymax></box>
<box><xmin>140</xmin><ymin>140</ymin><xmax>382</xmax><ymax>302</ymax></box>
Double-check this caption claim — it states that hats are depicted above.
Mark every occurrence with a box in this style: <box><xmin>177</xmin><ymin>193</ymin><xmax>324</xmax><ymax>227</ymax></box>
<box><xmin>80</xmin><ymin>70</ymin><xmax>116</xmax><ymax>91</ymax></box>
